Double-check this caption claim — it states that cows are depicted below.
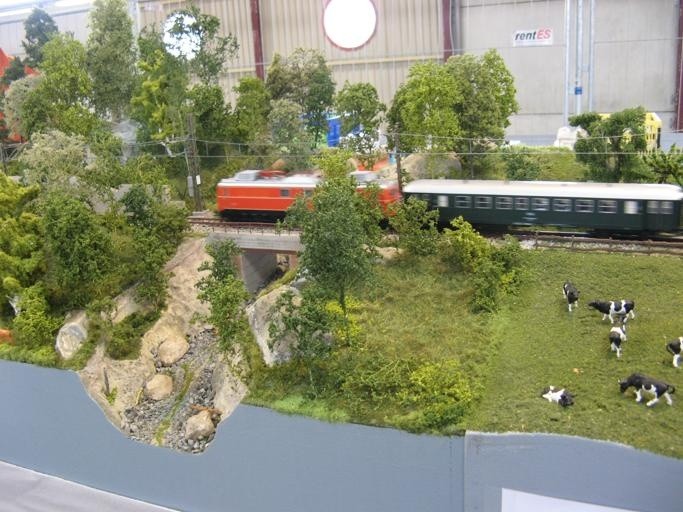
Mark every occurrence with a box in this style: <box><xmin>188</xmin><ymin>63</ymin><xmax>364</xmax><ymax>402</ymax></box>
<box><xmin>540</xmin><ymin>385</ymin><xmax>578</xmax><ymax>407</ymax></box>
<box><xmin>665</xmin><ymin>337</ymin><xmax>683</xmax><ymax>368</ymax></box>
<box><xmin>609</xmin><ymin>314</ymin><xmax>627</xmax><ymax>358</ymax></box>
<box><xmin>562</xmin><ymin>281</ymin><xmax>581</xmax><ymax>312</ymax></box>
<box><xmin>588</xmin><ymin>298</ymin><xmax>635</xmax><ymax>325</ymax></box>
<box><xmin>617</xmin><ymin>372</ymin><xmax>676</xmax><ymax>407</ymax></box>
